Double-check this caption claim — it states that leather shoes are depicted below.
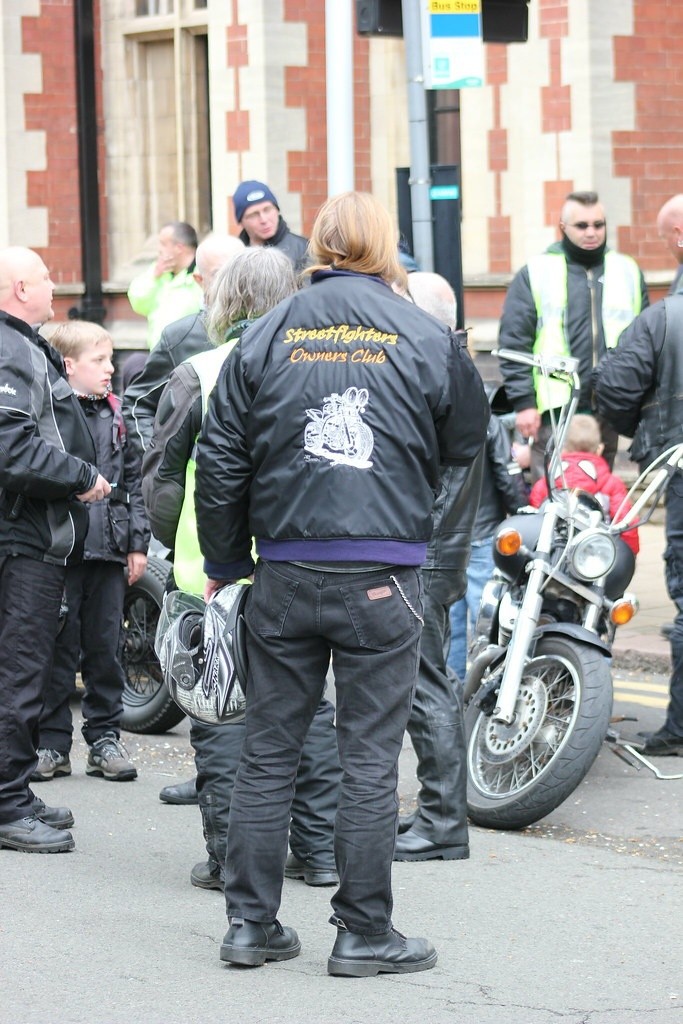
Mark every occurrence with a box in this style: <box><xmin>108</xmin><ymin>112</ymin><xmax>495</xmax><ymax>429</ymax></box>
<box><xmin>0</xmin><ymin>814</ymin><xmax>75</xmax><ymax>854</ymax></box>
<box><xmin>326</xmin><ymin>915</ymin><xmax>437</xmax><ymax>978</ymax></box>
<box><xmin>636</xmin><ymin>725</ymin><xmax>683</xmax><ymax>757</ymax></box>
<box><xmin>159</xmin><ymin>776</ymin><xmax>199</xmax><ymax>805</ymax></box>
<box><xmin>30</xmin><ymin>796</ymin><xmax>74</xmax><ymax>829</ymax></box>
<box><xmin>191</xmin><ymin>861</ymin><xmax>224</xmax><ymax>893</ymax></box>
<box><xmin>393</xmin><ymin>830</ymin><xmax>469</xmax><ymax>862</ymax></box>
<box><xmin>219</xmin><ymin>916</ymin><xmax>301</xmax><ymax>966</ymax></box>
<box><xmin>284</xmin><ymin>851</ymin><xmax>339</xmax><ymax>886</ymax></box>
<box><xmin>396</xmin><ymin>809</ymin><xmax>418</xmax><ymax>833</ymax></box>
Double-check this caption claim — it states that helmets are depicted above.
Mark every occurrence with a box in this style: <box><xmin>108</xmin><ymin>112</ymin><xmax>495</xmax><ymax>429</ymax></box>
<box><xmin>160</xmin><ymin>581</ymin><xmax>252</xmax><ymax>727</ymax></box>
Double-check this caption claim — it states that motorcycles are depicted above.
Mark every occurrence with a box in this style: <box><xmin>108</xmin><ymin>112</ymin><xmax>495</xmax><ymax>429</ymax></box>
<box><xmin>465</xmin><ymin>348</ymin><xmax>683</xmax><ymax>829</ymax></box>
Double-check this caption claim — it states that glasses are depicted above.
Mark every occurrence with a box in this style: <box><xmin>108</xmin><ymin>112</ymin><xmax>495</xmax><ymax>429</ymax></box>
<box><xmin>566</xmin><ymin>220</ymin><xmax>605</xmax><ymax>230</ymax></box>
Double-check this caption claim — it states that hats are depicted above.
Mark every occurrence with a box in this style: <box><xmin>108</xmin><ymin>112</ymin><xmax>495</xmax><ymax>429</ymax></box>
<box><xmin>233</xmin><ymin>181</ymin><xmax>279</xmax><ymax>223</ymax></box>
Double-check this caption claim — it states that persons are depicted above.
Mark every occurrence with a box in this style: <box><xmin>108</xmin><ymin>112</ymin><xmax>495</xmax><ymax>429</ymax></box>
<box><xmin>589</xmin><ymin>194</ymin><xmax>683</xmax><ymax>754</ymax></box>
<box><xmin>125</xmin><ymin>179</ymin><xmax>343</xmax><ymax>890</ymax></box>
<box><xmin>500</xmin><ymin>191</ymin><xmax>654</xmax><ymax>481</ymax></box>
<box><xmin>0</xmin><ymin>243</ymin><xmax>112</xmax><ymax>851</ymax></box>
<box><xmin>530</xmin><ymin>417</ymin><xmax>640</xmax><ymax>554</ymax></box>
<box><xmin>390</xmin><ymin>269</ymin><xmax>490</xmax><ymax>858</ymax></box>
<box><xmin>24</xmin><ymin>322</ymin><xmax>149</xmax><ymax>782</ymax></box>
<box><xmin>193</xmin><ymin>192</ymin><xmax>491</xmax><ymax>958</ymax></box>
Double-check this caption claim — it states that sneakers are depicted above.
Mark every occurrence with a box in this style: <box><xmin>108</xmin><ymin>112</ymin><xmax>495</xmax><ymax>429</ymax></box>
<box><xmin>30</xmin><ymin>748</ymin><xmax>71</xmax><ymax>781</ymax></box>
<box><xmin>85</xmin><ymin>732</ymin><xmax>137</xmax><ymax>782</ymax></box>
